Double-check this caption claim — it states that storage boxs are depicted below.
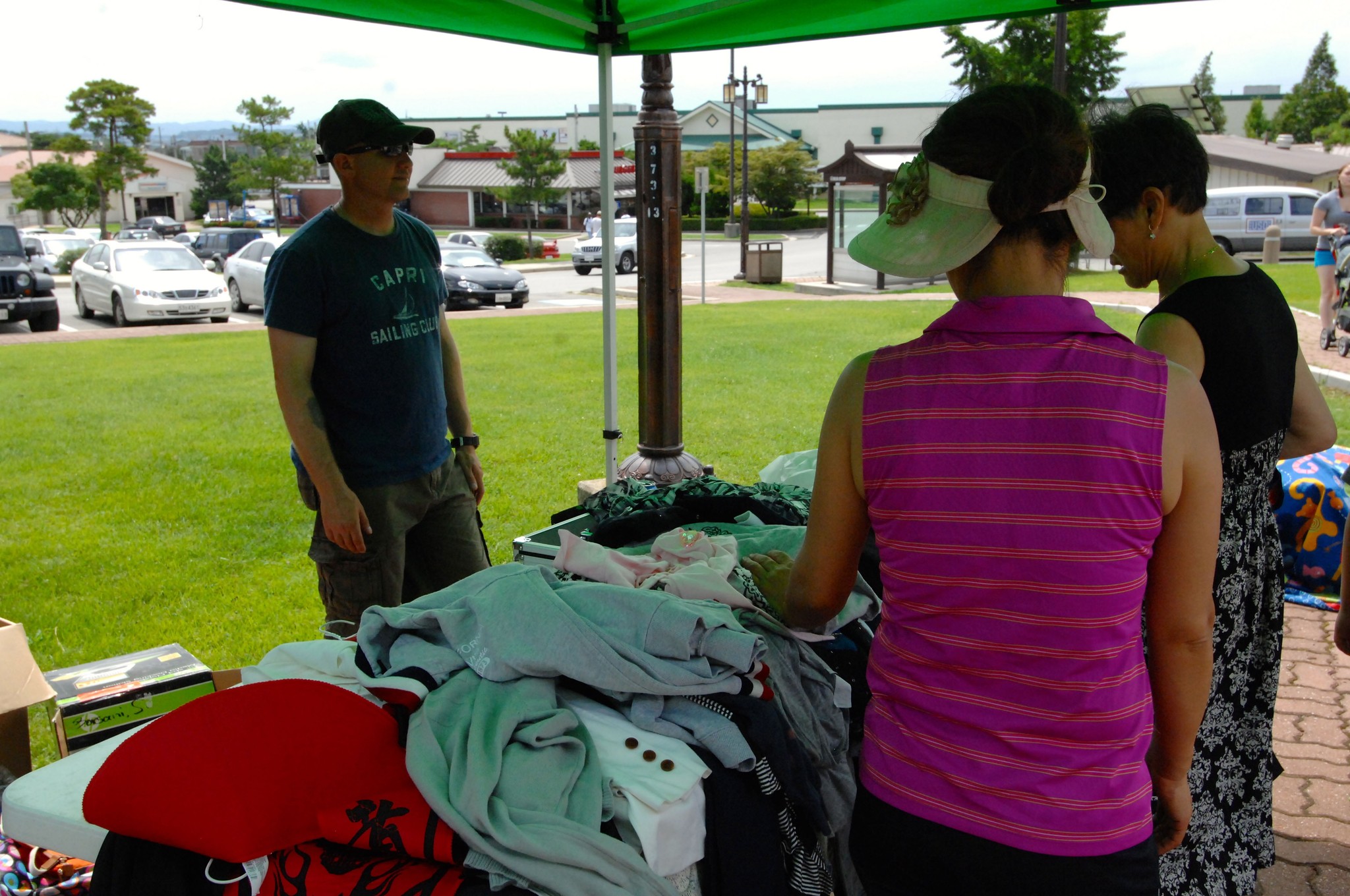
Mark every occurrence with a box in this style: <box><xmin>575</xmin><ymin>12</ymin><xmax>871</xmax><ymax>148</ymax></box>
<box><xmin>513</xmin><ymin>512</ymin><xmax>595</xmax><ymax>569</ymax></box>
<box><xmin>0</xmin><ymin>617</ymin><xmax>241</xmax><ymax>776</ymax></box>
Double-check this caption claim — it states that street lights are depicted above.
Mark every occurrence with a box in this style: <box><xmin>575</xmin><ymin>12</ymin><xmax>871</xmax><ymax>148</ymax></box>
<box><xmin>722</xmin><ymin>66</ymin><xmax>768</xmax><ymax>281</ymax></box>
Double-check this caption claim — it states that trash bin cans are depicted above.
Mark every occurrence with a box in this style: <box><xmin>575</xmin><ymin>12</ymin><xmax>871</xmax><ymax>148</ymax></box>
<box><xmin>745</xmin><ymin>241</ymin><xmax>783</xmax><ymax>284</ymax></box>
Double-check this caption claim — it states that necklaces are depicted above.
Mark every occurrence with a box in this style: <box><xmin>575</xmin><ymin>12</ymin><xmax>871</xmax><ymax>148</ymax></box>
<box><xmin>1158</xmin><ymin>244</ymin><xmax>1221</xmax><ymax>303</ymax></box>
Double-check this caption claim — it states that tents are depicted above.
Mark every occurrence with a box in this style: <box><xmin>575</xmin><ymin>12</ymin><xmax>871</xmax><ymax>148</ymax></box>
<box><xmin>225</xmin><ymin>0</ymin><xmax>1208</xmax><ymax>490</ymax></box>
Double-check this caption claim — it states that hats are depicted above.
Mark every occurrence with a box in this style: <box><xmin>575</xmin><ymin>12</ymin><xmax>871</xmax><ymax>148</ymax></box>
<box><xmin>847</xmin><ymin>144</ymin><xmax>1115</xmax><ymax>278</ymax></box>
<box><xmin>315</xmin><ymin>99</ymin><xmax>435</xmax><ymax>164</ymax></box>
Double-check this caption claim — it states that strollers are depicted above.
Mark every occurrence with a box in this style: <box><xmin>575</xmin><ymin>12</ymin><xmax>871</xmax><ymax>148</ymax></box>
<box><xmin>1319</xmin><ymin>228</ymin><xmax>1350</xmax><ymax>358</ymax></box>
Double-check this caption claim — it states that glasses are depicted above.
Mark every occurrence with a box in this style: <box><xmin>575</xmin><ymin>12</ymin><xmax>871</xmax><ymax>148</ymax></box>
<box><xmin>341</xmin><ymin>140</ymin><xmax>415</xmax><ymax>159</ymax></box>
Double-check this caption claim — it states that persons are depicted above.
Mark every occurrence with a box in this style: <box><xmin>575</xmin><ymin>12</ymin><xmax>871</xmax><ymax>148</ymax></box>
<box><xmin>1082</xmin><ymin>97</ymin><xmax>1338</xmax><ymax>896</ymax></box>
<box><xmin>620</xmin><ymin>210</ymin><xmax>630</xmax><ymax>219</ymax></box>
<box><xmin>264</xmin><ymin>99</ymin><xmax>492</xmax><ymax>642</ymax></box>
<box><xmin>1308</xmin><ymin>162</ymin><xmax>1350</xmax><ymax>346</ymax></box>
<box><xmin>743</xmin><ymin>85</ymin><xmax>1224</xmax><ymax>896</ymax></box>
<box><xmin>590</xmin><ymin>210</ymin><xmax>601</xmax><ymax>234</ymax></box>
<box><xmin>581</xmin><ymin>212</ymin><xmax>593</xmax><ymax>239</ymax></box>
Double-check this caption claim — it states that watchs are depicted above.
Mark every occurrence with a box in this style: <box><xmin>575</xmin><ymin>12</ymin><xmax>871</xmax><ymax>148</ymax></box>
<box><xmin>450</xmin><ymin>433</ymin><xmax>479</xmax><ymax>449</ymax></box>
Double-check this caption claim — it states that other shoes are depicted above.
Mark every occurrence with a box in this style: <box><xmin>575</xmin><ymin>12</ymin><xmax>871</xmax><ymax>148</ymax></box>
<box><xmin>1329</xmin><ymin>330</ymin><xmax>1337</xmax><ymax>342</ymax></box>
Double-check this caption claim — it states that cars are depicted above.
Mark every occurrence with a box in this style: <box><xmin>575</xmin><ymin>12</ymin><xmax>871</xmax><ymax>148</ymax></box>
<box><xmin>435</xmin><ymin>241</ymin><xmax>530</xmax><ymax>311</ymax></box>
<box><xmin>71</xmin><ymin>239</ymin><xmax>233</xmax><ymax>329</ymax></box>
<box><xmin>224</xmin><ymin>235</ymin><xmax>293</xmax><ymax>313</ymax></box>
<box><xmin>446</xmin><ymin>230</ymin><xmax>492</xmax><ymax>253</ymax></box>
<box><xmin>12</xmin><ymin>214</ymin><xmax>202</xmax><ymax>274</ymax></box>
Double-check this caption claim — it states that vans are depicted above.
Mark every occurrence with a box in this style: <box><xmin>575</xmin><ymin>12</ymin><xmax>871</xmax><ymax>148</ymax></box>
<box><xmin>1202</xmin><ymin>185</ymin><xmax>1325</xmax><ymax>257</ymax></box>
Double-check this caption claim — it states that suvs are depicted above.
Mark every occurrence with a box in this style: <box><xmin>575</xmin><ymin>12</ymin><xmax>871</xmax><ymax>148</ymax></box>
<box><xmin>0</xmin><ymin>224</ymin><xmax>61</xmax><ymax>331</ymax></box>
<box><xmin>570</xmin><ymin>217</ymin><xmax>639</xmax><ymax>275</ymax></box>
<box><xmin>186</xmin><ymin>228</ymin><xmax>264</xmax><ymax>275</ymax></box>
